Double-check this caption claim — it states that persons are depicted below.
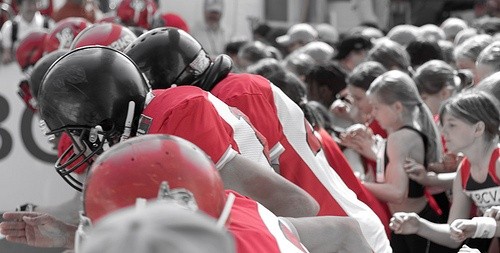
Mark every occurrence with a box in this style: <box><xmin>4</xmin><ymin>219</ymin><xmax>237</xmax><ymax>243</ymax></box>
<box><xmin>0</xmin><ymin>0</ymin><xmax>190</xmax><ymax>64</ymax></box>
<box><xmin>188</xmin><ymin>0</ymin><xmax>261</xmax><ymax>61</ymax></box>
<box><xmin>352</xmin><ymin>17</ymin><xmax>500</xmax><ymax>253</ymax></box>
<box><xmin>0</xmin><ymin>18</ymin><xmax>393</xmax><ymax>253</ymax></box>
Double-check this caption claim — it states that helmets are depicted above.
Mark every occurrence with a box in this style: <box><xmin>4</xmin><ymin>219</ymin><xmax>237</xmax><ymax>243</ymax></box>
<box><xmin>122</xmin><ymin>24</ymin><xmax>215</xmax><ymax>92</ymax></box>
<box><xmin>48</xmin><ymin>16</ymin><xmax>90</xmax><ymax>50</ymax></box>
<box><xmin>83</xmin><ymin>133</ymin><xmax>227</xmax><ymax>224</ymax></box>
<box><xmin>69</xmin><ymin>21</ymin><xmax>137</xmax><ymax>54</ymax></box>
<box><xmin>15</xmin><ymin>32</ymin><xmax>59</xmax><ymax>68</ymax></box>
<box><xmin>38</xmin><ymin>44</ymin><xmax>155</xmax><ymax>156</ymax></box>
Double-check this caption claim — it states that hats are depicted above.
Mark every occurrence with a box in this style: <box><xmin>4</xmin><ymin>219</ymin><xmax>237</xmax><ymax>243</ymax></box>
<box><xmin>78</xmin><ymin>197</ymin><xmax>238</xmax><ymax>253</ymax></box>
<box><xmin>275</xmin><ymin>23</ymin><xmax>319</xmax><ymax>47</ymax></box>
<box><xmin>390</xmin><ymin>17</ymin><xmax>468</xmax><ymax>48</ymax></box>
